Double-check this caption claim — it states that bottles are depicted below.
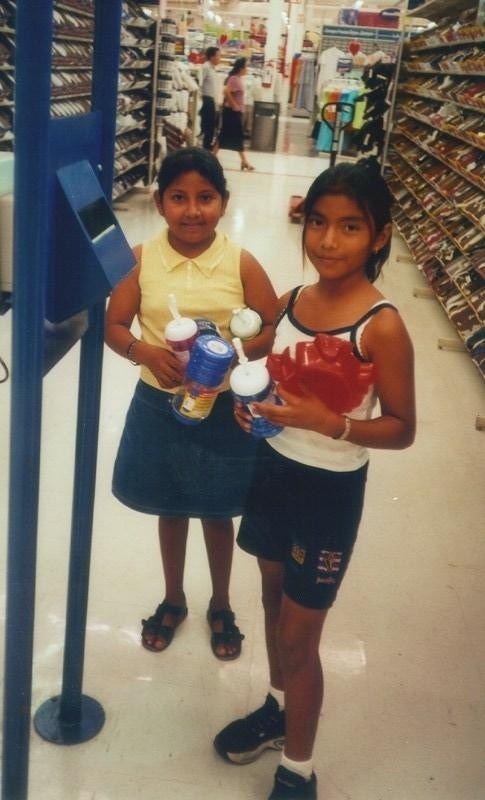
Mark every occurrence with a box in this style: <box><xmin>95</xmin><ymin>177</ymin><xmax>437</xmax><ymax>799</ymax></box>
<box><xmin>229</xmin><ymin>308</ymin><xmax>263</xmax><ymax>342</ymax></box>
<box><xmin>163</xmin><ymin>317</ymin><xmax>235</xmax><ymax>427</ymax></box>
<box><xmin>229</xmin><ymin>362</ymin><xmax>285</xmax><ymax>438</ymax></box>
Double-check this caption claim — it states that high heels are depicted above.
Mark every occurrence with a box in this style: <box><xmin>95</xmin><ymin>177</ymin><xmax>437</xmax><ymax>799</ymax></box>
<box><xmin>241</xmin><ymin>161</ymin><xmax>255</xmax><ymax>171</ymax></box>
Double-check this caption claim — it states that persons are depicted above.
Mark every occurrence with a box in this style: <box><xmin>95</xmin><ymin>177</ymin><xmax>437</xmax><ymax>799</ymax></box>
<box><xmin>210</xmin><ymin>160</ymin><xmax>417</xmax><ymax>800</ymax></box>
<box><xmin>201</xmin><ymin>47</ymin><xmax>221</xmax><ymax>150</ymax></box>
<box><xmin>211</xmin><ymin>57</ymin><xmax>256</xmax><ymax>172</ymax></box>
<box><xmin>101</xmin><ymin>146</ymin><xmax>278</xmax><ymax>662</ymax></box>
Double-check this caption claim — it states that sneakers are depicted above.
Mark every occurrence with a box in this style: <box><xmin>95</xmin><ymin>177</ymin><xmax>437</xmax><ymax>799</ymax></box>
<box><xmin>266</xmin><ymin>764</ymin><xmax>317</xmax><ymax>800</ymax></box>
<box><xmin>214</xmin><ymin>691</ymin><xmax>285</xmax><ymax>765</ymax></box>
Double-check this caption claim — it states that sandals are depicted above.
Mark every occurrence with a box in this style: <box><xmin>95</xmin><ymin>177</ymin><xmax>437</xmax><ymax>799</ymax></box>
<box><xmin>141</xmin><ymin>600</ymin><xmax>188</xmax><ymax>652</ymax></box>
<box><xmin>207</xmin><ymin>609</ymin><xmax>244</xmax><ymax>661</ymax></box>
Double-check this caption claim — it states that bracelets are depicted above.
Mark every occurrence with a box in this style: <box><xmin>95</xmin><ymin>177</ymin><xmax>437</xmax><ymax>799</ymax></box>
<box><xmin>333</xmin><ymin>416</ymin><xmax>352</xmax><ymax>441</ymax></box>
<box><xmin>126</xmin><ymin>339</ymin><xmax>141</xmax><ymax>367</ymax></box>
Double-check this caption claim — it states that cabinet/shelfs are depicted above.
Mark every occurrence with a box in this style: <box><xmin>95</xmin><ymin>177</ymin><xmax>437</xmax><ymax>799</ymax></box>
<box><xmin>380</xmin><ymin>0</ymin><xmax>485</xmax><ymax>430</ymax></box>
<box><xmin>0</xmin><ymin>0</ymin><xmax>163</xmax><ymax>201</ymax></box>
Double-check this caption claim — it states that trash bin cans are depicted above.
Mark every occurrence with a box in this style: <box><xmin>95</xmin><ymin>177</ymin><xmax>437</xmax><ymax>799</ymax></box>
<box><xmin>250</xmin><ymin>100</ymin><xmax>279</xmax><ymax>152</ymax></box>
